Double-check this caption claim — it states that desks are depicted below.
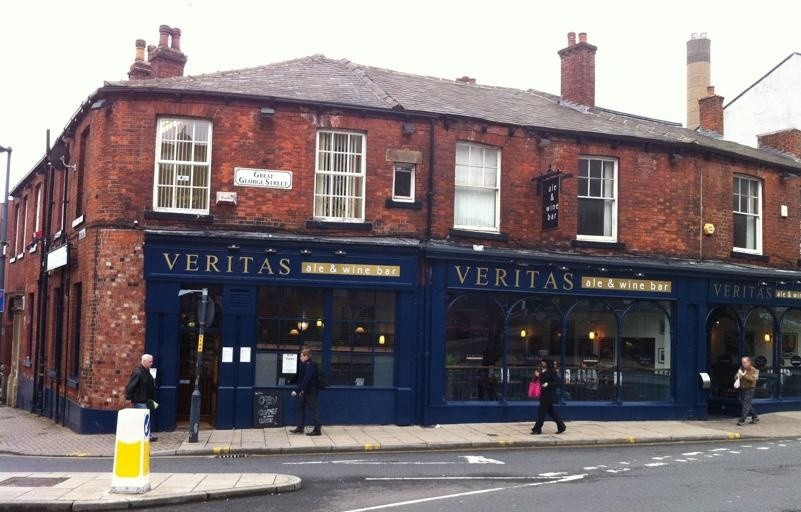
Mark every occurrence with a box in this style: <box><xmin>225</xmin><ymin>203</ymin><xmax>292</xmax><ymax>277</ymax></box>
<box><xmin>568</xmin><ymin>381</ymin><xmax>595</xmax><ymax>401</ymax></box>
<box><xmin>498</xmin><ymin>380</ymin><xmax>522</xmax><ymax>401</ymax></box>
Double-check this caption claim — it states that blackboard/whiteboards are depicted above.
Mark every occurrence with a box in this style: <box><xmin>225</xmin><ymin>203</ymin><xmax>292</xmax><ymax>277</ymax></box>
<box><xmin>251</xmin><ymin>386</ymin><xmax>284</xmax><ymax>428</ymax></box>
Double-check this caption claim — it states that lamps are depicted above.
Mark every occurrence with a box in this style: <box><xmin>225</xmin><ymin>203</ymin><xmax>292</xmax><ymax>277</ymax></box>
<box><xmin>443</xmin><ymin>119</ymin><xmax>450</xmax><ymax>130</ymax></box>
<box><xmin>14</xmin><ymin>99</ymin><xmax>107</xmax><ymax>191</ymax></box>
<box><xmin>639</xmin><ymin>143</ymin><xmax>649</xmax><ymax>157</ymax></box>
<box><xmin>402</xmin><ymin>122</ymin><xmax>417</xmax><ymax>136</ymax></box>
<box><xmin>588</xmin><ymin>330</ymin><xmax>598</xmax><ymax>340</ymax></box>
<box><xmin>356</xmin><ymin>327</ymin><xmax>364</xmax><ymax>334</ymax></box>
<box><xmin>779</xmin><ymin>168</ymin><xmax>796</xmax><ymax>180</ymax></box>
<box><xmin>260</xmin><ymin>108</ymin><xmax>275</xmax><ymax>119</ymax></box>
<box><xmin>520</xmin><ymin>329</ymin><xmax>528</xmax><ymax>338</ymax></box>
<box><xmin>608</xmin><ymin>139</ymin><xmax>617</xmax><ymax>150</ymax></box>
<box><xmin>481</xmin><ymin>126</ymin><xmax>487</xmax><ymax>135</ymax></box>
<box><xmin>506</xmin><ymin>124</ymin><xmax>522</xmax><ymax>139</ymax></box>
<box><xmin>316</xmin><ymin>318</ymin><xmax>324</xmax><ymax>327</ymax></box>
<box><xmin>668</xmin><ymin>152</ymin><xmax>685</xmax><ymax>165</ymax></box>
<box><xmin>764</xmin><ymin>332</ymin><xmax>772</xmax><ymax>342</ymax></box>
<box><xmin>524</xmin><ymin>128</ymin><xmax>546</xmax><ymax>149</ymax></box>
<box><xmin>575</xmin><ymin>133</ymin><xmax>582</xmax><ymax>146</ymax></box>
<box><xmin>297</xmin><ymin>319</ymin><xmax>309</xmax><ymax>331</ymax></box>
<box><xmin>289</xmin><ymin>329</ymin><xmax>299</xmax><ymax>336</ymax></box>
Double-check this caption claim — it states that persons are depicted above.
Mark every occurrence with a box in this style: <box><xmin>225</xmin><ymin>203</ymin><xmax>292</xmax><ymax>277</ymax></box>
<box><xmin>476</xmin><ymin>371</ymin><xmax>501</xmax><ymax>402</ymax></box>
<box><xmin>529</xmin><ymin>357</ymin><xmax>567</xmax><ymax>436</ymax></box>
<box><xmin>125</xmin><ymin>353</ymin><xmax>160</xmax><ymax>442</ymax></box>
<box><xmin>290</xmin><ymin>351</ymin><xmax>323</xmax><ymax>435</ymax></box>
<box><xmin>734</xmin><ymin>356</ymin><xmax>763</xmax><ymax>425</ymax></box>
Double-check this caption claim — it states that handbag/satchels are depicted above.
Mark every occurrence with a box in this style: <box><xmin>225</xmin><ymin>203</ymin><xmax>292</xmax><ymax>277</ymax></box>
<box><xmin>529</xmin><ymin>382</ymin><xmax>542</xmax><ymax>399</ymax></box>
<box><xmin>148</xmin><ymin>399</ymin><xmax>159</xmax><ymax>411</ymax></box>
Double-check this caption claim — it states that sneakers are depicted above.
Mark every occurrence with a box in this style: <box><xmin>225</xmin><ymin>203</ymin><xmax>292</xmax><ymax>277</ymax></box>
<box><xmin>737</xmin><ymin>418</ymin><xmax>760</xmax><ymax>426</ymax></box>
<box><xmin>290</xmin><ymin>428</ymin><xmax>304</xmax><ymax>433</ymax></box>
<box><xmin>150</xmin><ymin>434</ymin><xmax>156</xmax><ymax>442</ymax></box>
<box><xmin>530</xmin><ymin>427</ymin><xmax>566</xmax><ymax>434</ymax></box>
<box><xmin>307</xmin><ymin>431</ymin><xmax>321</xmax><ymax>436</ymax></box>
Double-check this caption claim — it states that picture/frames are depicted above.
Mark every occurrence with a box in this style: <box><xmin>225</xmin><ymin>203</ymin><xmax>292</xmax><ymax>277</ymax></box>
<box><xmin>597</xmin><ymin>337</ymin><xmax>615</xmax><ymax>364</ymax></box>
<box><xmin>658</xmin><ymin>348</ymin><xmax>664</xmax><ymax>363</ymax></box>
<box><xmin>781</xmin><ymin>332</ymin><xmax>798</xmax><ymax>358</ymax></box>
<box><xmin>724</xmin><ymin>331</ymin><xmax>754</xmax><ymax>356</ymax></box>
<box><xmin>576</xmin><ymin>335</ymin><xmax>592</xmax><ymax>360</ymax></box>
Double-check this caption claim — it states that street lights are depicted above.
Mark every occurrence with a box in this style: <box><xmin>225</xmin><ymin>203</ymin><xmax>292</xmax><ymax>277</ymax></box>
<box><xmin>177</xmin><ymin>285</ymin><xmax>211</xmax><ymax>445</ymax></box>
<box><xmin>0</xmin><ymin>144</ymin><xmax>13</xmax><ymax>329</ymax></box>
